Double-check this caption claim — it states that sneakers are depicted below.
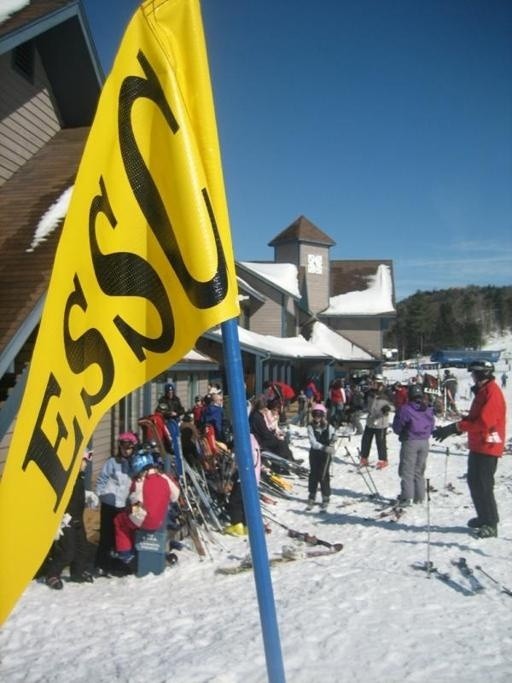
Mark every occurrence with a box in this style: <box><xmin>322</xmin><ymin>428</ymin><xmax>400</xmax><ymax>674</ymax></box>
<box><xmin>44</xmin><ymin>574</ymin><xmax>64</xmax><ymax>590</ymax></box>
<box><xmin>71</xmin><ymin>564</ymin><xmax>109</xmax><ymax>584</ymax></box>
<box><xmin>292</xmin><ymin>458</ymin><xmax>304</xmax><ymax>466</ymax></box>
<box><xmin>478</xmin><ymin>526</ymin><xmax>498</xmax><ymax>538</ymax></box>
<box><xmin>467</xmin><ymin>517</ymin><xmax>493</xmax><ymax>528</ymax></box>
<box><xmin>307</xmin><ymin>495</ymin><xmax>330</xmax><ymax>506</ymax></box>
<box><xmin>397</xmin><ymin>494</ymin><xmax>426</xmax><ymax>505</ymax></box>
<box><xmin>225</xmin><ymin>522</ymin><xmax>249</xmax><ymax>536</ymax></box>
<box><xmin>107</xmin><ymin>549</ymin><xmax>135</xmax><ymax>565</ymax></box>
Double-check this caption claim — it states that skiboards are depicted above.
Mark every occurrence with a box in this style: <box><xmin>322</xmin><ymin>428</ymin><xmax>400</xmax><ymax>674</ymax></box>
<box><xmin>215</xmin><ymin>440</ymin><xmax>312</xmax><ymax>518</ymax></box>
<box><xmin>218</xmin><ymin>520</ymin><xmax>342</xmax><ymax>575</ymax></box>
<box><xmin>142</xmin><ymin>413</ymin><xmax>233</xmax><ymax>559</ymax></box>
<box><xmin>424</xmin><ymin>555</ymin><xmax>489</xmax><ymax>596</ymax></box>
<box><xmin>367</xmin><ymin>505</ymin><xmax>406</xmax><ymax>522</ymax></box>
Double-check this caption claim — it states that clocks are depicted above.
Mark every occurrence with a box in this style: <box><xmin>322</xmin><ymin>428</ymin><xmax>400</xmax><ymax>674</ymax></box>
<box><xmin>307</xmin><ymin>254</ymin><xmax>323</xmax><ymax>274</ymax></box>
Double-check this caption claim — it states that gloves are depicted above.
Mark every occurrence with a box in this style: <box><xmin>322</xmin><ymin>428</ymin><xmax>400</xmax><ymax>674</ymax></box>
<box><xmin>429</xmin><ymin>422</ymin><xmax>462</xmax><ymax>443</ymax></box>
<box><xmin>381</xmin><ymin>405</ymin><xmax>390</xmax><ymax>416</ymax></box>
<box><xmin>328</xmin><ymin>434</ymin><xmax>338</xmax><ymax>445</ymax></box>
<box><xmin>321</xmin><ymin>446</ymin><xmax>337</xmax><ymax>455</ymax></box>
<box><xmin>85</xmin><ymin>489</ymin><xmax>99</xmax><ymax>511</ymax></box>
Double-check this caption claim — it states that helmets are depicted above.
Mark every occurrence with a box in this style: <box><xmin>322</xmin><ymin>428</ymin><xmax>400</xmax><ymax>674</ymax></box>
<box><xmin>155</xmin><ymin>384</ymin><xmax>194</xmax><ymax>424</ymax></box>
<box><xmin>407</xmin><ymin>385</ymin><xmax>424</xmax><ymax>400</ymax></box>
<box><xmin>118</xmin><ymin>431</ymin><xmax>138</xmax><ymax>446</ymax></box>
<box><xmin>127</xmin><ymin>448</ymin><xmax>155</xmax><ymax>480</ymax></box>
<box><xmin>373</xmin><ymin>373</ymin><xmax>385</xmax><ymax>384</ymax></box>
<box><xmin>466</xmin><ymin>359</ymin><xmax>496</xmax><ymax>375</ymax></box>
<box><xmin>310</xmin><ymin>403</ymin><xmax>328</xmax><ymax>415</ymax></box>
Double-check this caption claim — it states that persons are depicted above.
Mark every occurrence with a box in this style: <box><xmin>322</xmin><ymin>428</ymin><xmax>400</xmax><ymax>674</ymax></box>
<box><xmin>299</xmin><ymin>368</ymin><xmax>390</xmax><ymax>468</ymax></box>
<box><xmin>43</xmin><ymin>368</ymin><xmax>299</xmax><ymax>588</ymax></box>
<box><xmin>392</xmin><ymin>385</ymin><xmax>435</xmax><ymax>506</ymax></box>
<box><xmin>390</xmin><ymin>369</ymin><xmax>458</xmax><ymax>411</ymax></box>
<box><xmin>305</xmin><ymin>404</ymin><xmax>338</xmax><ymax>513</ymax></box>
<box><xmin>433</xmin><ymin>358</ymin><xmax>506</xmax><ymax>537</ymax></box>
